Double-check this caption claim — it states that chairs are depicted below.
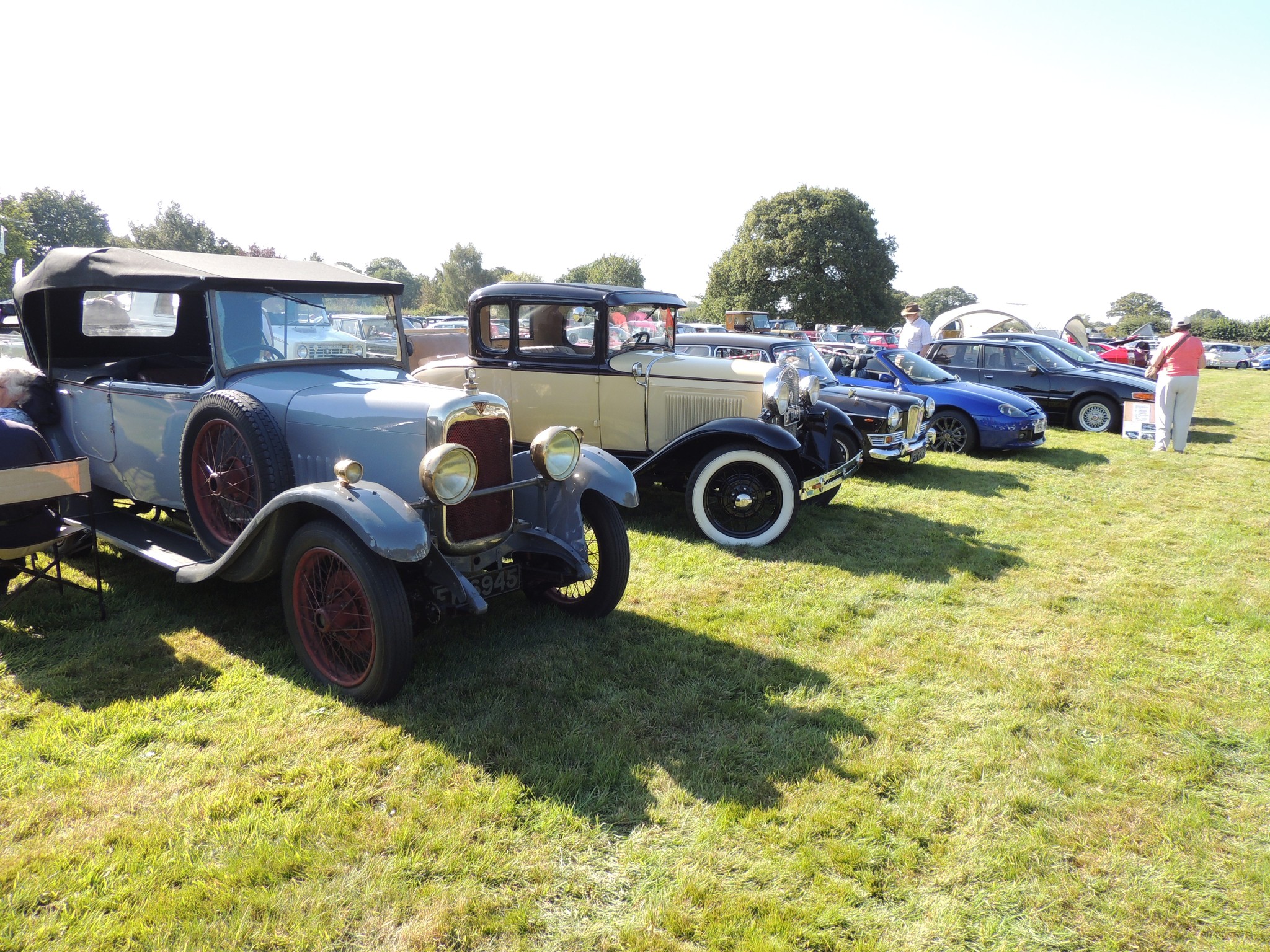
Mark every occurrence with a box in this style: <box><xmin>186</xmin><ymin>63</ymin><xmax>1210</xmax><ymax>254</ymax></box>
<box><xmin>0</xmin><ymin>453</ymin><xmax>110</xmax><ymax>635</ymax></box>
<box><xmin>850</xmin><ymin>355</ymin><xmax>871</xmax><ymax>379</ymax></box>
<box><xmin>827</xmin><ymin>357</ymin><xmax>845</xmax><ymax>376</ymax></box>
<box><xmin>989</xmin><ymin>353</ymin><xmax>1004</xmax><ymax>369</ymax></box>
<box><xmin>983</xmin><ymin>353</ymin><xmax>992</xmax><ymax>369</ymax></box>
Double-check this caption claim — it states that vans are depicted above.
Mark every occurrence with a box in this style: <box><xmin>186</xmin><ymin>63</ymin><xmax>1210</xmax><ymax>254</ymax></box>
<box><xmin>111</xmin><ymin>291</ymin><xmax>366</xmax><ymax>362</ymax></box>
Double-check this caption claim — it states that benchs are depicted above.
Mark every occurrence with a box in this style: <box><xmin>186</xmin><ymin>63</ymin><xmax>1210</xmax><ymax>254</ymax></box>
<box><xmin>97</xmin><ymin>355</ymin><xmax>214</xmax><ymax>388</ymax></box>
<box><xmin>509</xmin><ymin>346</ymin><xmax>576</xmax><ymax>356</ymax></box>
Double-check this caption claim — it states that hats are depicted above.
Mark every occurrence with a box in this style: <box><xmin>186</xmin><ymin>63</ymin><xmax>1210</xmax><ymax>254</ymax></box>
<box><xmin>1008</xmin><ymin>327</ymin><xmax>1016</xmax><ymax>332</ymax></box>
<box><xmin>1172</xmin><ymin>315</ymin><xmax>1190</xmax><ymax>326</ymax></box>
<box><xmin>901</xmin><ymin>303</ymin><xmax>924</xmax><ymax>316</ymax></box>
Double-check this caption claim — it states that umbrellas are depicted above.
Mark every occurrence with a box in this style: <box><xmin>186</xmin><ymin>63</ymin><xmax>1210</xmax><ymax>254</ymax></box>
<box><xmin>627</xmin><ymin>311</ymin><xmax>658</xmax><ymax>331</ymax></box>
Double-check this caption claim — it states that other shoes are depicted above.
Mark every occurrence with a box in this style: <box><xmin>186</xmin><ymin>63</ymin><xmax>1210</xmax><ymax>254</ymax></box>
<box><xmin>1151</xmin><ymin>447</ymin><xmax>1166</xmax><ymax>452</ymax></box>
<box><xmin>1173</xmin><ymin>450</ymin><xmax>1183</xmax><ymax>454</ymax></box>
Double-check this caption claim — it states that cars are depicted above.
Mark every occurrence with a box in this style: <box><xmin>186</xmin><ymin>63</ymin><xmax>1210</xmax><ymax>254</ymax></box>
<box><xmin>7</xmin><ymin>242</ymin><xmax>639</xmax><ymax>701</ymax></box>
<box><xmin>1243</xmin><ymin>346</ymin><xmax>1255</xmax><ymax>357</ymax></box>
<box><xmin>1201</xmin><ymin>343</ymin><xmax>1250</xmax><ymax>370</ymax></box>
<box><xmin>827</xmin><ymin>349</ymin><xmax>1047</xmax><ymax>456</ymax></box>
<box><xmin>419</xmin><ymin>316</ymin><xmax>530</xmax><ymax>340</ymax></box>
<box><xmin>725</xmin><ymin>310</ymin><xmax>900</xmax><ymax>358</ymax></box>
<box><xmin>640</xmin><ymin>333</ymin><xmax>936</xmax><ymax>466</ymax></box>
<box><xmin>1254</xmin><ymin>345</ymin><xmax>1270</xmax><ymax>357</ymax></box>
<box><xmin>408</xmin><ymin>280</ymin><xmax>865</xmax><ymax>549</ymax></box>
<box><xmin>1250</xmin><ymin>354</ymin><xmax>1270</xmax><ymax>371</ymax></box>
<box><xmin>566</xmin><ymin>320</ymin><xmax>726</xmax><ymax>352</ymax></box>
<box><xmin>316</xmin><ymin>314</ymin><xmax>418</xmax><ymax>352</ymax></box>
<box><xmin>1070</xmin><ymin>333</ymin><xmax>1153</xmax><ymax>367</ymax></box>
<box><xmin>922</xmin><ymin>339</ymin><xmax>1155</xmax><ymax>434</ymax></box>
<box><xmin>967</xmin><ymin>333</ymin><xmax>1155</xmax><ymax>386</ymax></box>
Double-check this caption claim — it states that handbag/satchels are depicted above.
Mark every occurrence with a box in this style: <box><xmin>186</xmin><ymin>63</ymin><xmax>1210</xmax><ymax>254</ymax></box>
<box><xmin>1145</xmin><ymin>365</ymin><xmax>1160</xmax><ymax>380</ymax></box>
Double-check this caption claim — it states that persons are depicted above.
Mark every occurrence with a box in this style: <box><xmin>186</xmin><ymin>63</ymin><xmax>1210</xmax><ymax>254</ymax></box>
<box><xmin>609</xmin><ymin>305</ymin><xmax>630</xmax><ymax>338</ymax></box>
<box><xmin>0</xmin><ymin>356</ymin><xmax>63</xmax><ymax>599</ymax></box>
<box><xmin>771</xmin><ymin>322</ymin><xmax>780</xmax><ymax>330</ymax></box>
<box><xmin>1150</xmin><ymin>314</ymin><xmax>1206</xmax><ymax>454</ymax></box>
<box><xmin>894</xmin><ymin>303</ymin><xmax>934</xmax><ymax>377</ymax></box>
<box><xmin>1006</xmin><ymin>327</ymin><xmax>1016</xmax><ymax>341</ymax></box>
<box><xmin>848</xmin><ymin>325</ymin><xmax>858</xmax><ymax>333</ymax></box>
<box><xmin>1135</xmin><ymin>341</ymin><xmax>1150</xmax><ymax>360</ymax></box>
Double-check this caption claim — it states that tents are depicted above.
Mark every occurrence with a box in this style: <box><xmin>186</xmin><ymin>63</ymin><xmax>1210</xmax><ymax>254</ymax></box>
<box><xmin>930</xmin><ymin>302</ymin><xmax>1089</xmax><ymax>353</ymax></box>
